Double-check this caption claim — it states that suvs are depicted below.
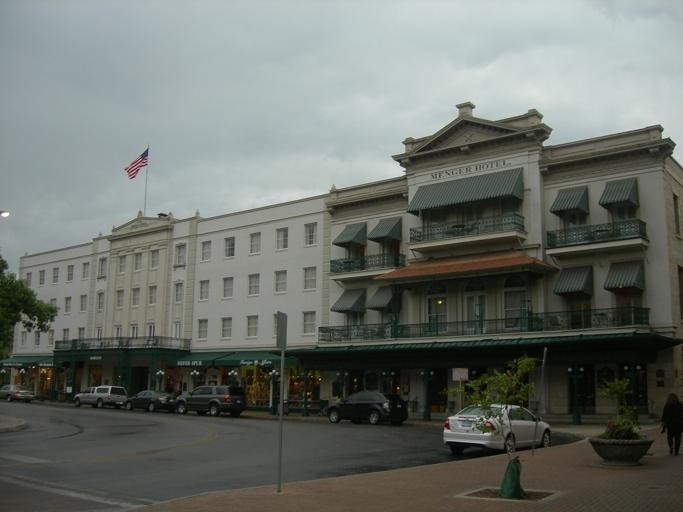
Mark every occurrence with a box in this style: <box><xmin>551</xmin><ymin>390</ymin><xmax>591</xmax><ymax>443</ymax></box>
<box><xmin>175</xmin><ymin>386</ymin><xmax>246</xmax><ymax>417</ymax></box>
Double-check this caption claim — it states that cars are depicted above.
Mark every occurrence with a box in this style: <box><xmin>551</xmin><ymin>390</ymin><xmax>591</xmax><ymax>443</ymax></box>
<box><xmin>323</xmin><ymin>387</ymin><xmax>408</xmax><ymax>426</ymax></box>
<box><xmin>0</xmin><ymin>384</ymin><xmax>36</xmax><ymax>402</ymax></box>
<box><xmin>442</xmin><ymin>402</ymin><xmax>553</xmax><ymax>455</ymax></box>
<box><xmin>126</xmin><ymin>390</ymin><xmax>176</xmax><ymax>413</ymax></box>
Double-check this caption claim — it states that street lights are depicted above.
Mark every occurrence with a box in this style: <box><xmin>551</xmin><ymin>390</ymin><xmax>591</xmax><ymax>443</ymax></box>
<box><xmin>567</xmin><ymin>365</ymin><xmax>584</xmax><ymax>424</ymax></box>
<box><xmin>40</xmin><ymin>368</ymin><xmax>46</xmax><ymax>402</ymax></box>
<box><xmin>292</xmin><ymin>369</ymin><xmax>322</xmax><ymax>417</ymax></box>
<box><xmin>419</xmin><ymin>370</ymin><xmax>436</xmax><ymax>420</ymax></box>
<box><xmin>190</xmin><ymin>369</ymin><xmax>200</xmax><ymax>388</ymax></box>
<box><xmin>624</xmin><ymin>363</ymin><xmax>642</xmax><ymax>425</ymax></box>
<box><xmin>20</xmin><ymin>367</ymin><xmax>26</xmax><ymax>385</ymax></box>
<box><xmin>0</xmin><ymin>368</ymin><xmax>7</xmax><ymax>385</ymax></box>
<box><xmin>266</xmin><ymin>369</ymin><xmax>281</xmax><ymax>416</ymax></box>
<box><xmin>228</xmin><ymin>369</ymin><xmax>238</xmax><ymax>385</ymax></box>
<box><xmin>156</xmin><ymin>368</ymin><xmax>165</xmax><ymax>391</ymax></box>
<box><xmin>335</xmin><ymin>370</ymin><xmax>350</xmax><ymax>398</ymax></box>
<box><xmin>382</xmin><ymin>368</ymin><xmax>397</xmax><ymax>394</ymax></box>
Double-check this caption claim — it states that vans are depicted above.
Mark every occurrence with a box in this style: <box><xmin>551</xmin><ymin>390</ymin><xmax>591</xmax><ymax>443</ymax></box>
<box><xmin>72</xmin><ymin>384</ymin><xmax>128</xmax><ymax>409</ymax></box>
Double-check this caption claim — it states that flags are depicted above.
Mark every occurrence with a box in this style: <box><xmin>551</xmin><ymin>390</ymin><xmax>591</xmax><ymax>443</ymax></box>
<box><xmin>124</xmin><ymin>147</ymin><xmax>149</xmax><ymax>179</ymax></box>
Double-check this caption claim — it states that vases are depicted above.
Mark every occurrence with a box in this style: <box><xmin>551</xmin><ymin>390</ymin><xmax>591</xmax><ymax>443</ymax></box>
<box><xmin>431</xmin><ymin>404</ymin><xmax>440</xmax><ymax>412</ymax></box>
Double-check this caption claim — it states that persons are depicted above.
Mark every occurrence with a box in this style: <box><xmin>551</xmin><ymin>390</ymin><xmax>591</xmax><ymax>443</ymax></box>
<box><xmin>662</xmin><ymin>393</ymin><xmax>683</xmax><ymax>456</ymax></box>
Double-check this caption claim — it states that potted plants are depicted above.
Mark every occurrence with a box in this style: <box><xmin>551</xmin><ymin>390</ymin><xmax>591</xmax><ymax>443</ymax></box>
<box><xmin>437</xmin><ymin>388</ymin><xmax>448</xmax><ymax>413</ymax></box>
<box><xmin>450</xmin><ymin>353</ymin><xmax>563</xmax><ymax>512</ymax></box>
<box><xmin>587</xmin><ymin>373</ymin><xmax>655</xmax><ymax>466</ymax></box>
